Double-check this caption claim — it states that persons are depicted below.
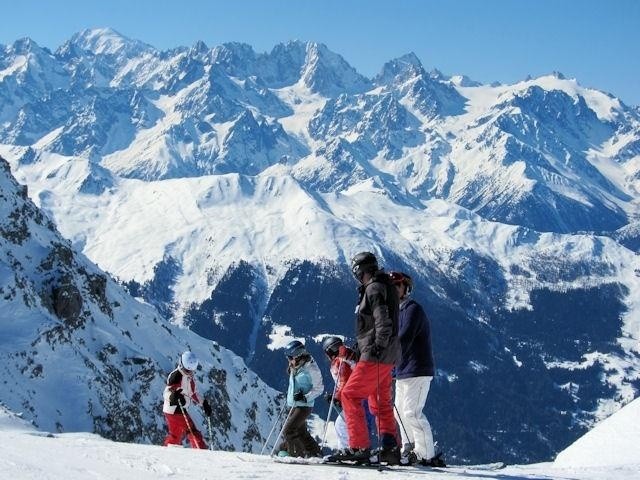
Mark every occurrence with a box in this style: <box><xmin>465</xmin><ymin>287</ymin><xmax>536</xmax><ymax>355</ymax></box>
<box><xmin>388</xmin><ymin>270</ymin><xmax>436</xmax><ymax>465</ymax></box>
<box><xmin>162</xmin><ymin>351</ymin><xmax>212</xmax><ymax>449</ymax></box>
<box><xmin>278</xmin><ymin>340</ymin><xmax>325</xmax><ymax>458</ymax></box>
<box><xmin>322</xmin><ymin>337</ymin><xmax>360</xmax><ymax>448</ymax></box>
<box><xmin>326</xmin><ymin>250</ymin><xmax>403</xmax><ymax>465</ymax></box>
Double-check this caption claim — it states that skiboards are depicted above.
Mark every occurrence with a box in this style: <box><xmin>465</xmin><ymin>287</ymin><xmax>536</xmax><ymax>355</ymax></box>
<box><xmin>237</xmin><ymin>452</ymin><xmax>506</xmax><ymax>473</ymax></box>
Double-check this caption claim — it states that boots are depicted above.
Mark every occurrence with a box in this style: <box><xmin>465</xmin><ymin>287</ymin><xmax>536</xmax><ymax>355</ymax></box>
<box><xmin>277</xmin><ymin>432</ymin><xmax>323</xmax><ymax>460</ymax></box>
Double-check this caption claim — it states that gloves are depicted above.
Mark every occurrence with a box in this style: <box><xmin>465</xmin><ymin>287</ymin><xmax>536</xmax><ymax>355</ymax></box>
<box><xmin>203</xmin><ymin>398</ymin><xmax>214</xmax><ymax>417</ymax></box>
<box><xmin>293</xmin><ymin>388</ymin><xmax>307</xmax><ymax>403</ymax></box>
<box><xmin>169</xmin><ymin>389</ymin><xmax>185</xmax><ymax>407</ymax></box>
<box><xmin>322</xmin><ymin>393</ymin><xmax>332</xmax><ymax>400</ymax></box>
<box><xmin>371</xmin><ymin>342</ymin><xmax>387</xmax><ymax>359</ymax></box>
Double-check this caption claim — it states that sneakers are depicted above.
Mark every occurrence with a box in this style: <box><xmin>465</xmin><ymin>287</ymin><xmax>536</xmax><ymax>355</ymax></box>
<box><xmin>327</xmin><ymin>433</ymin><xmax>443</xmax><ymax>468</ymax></box>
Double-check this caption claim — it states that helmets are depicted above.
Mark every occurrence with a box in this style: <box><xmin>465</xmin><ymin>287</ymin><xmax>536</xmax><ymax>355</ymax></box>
<box><xmin>322</xmin><ymin>337</ymin><xmax>342</xmax><ymax>354</ymax></box>
<box><xmin>284</xmin><ymin>340</ymin><xmax>305</xmax><ymax>361</ymax></box>
<box><xmin>388</xmin><ymin>270</ymin><xmax>413</xmax><ymax>298</ymax></box>
<box><xmin>351</xmin><ymin>251</ymin><xmax>378</xmax><ymax>283</ymax></box>
<box><xmin>178</xmin><ymin>351</ymin><xmax>200</xmax><ymax>373</ymax></box>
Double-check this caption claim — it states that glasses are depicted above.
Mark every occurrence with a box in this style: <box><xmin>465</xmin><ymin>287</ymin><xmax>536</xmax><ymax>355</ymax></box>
<box><xmin>287</xmin><ymin>356</ymin><xmax>296</xmax><ymax>361</ymax></box>
<box><xmin>389</xmin><ymin>272</ymin><xmax>412</xmax><ymax>286</ymax></box>
